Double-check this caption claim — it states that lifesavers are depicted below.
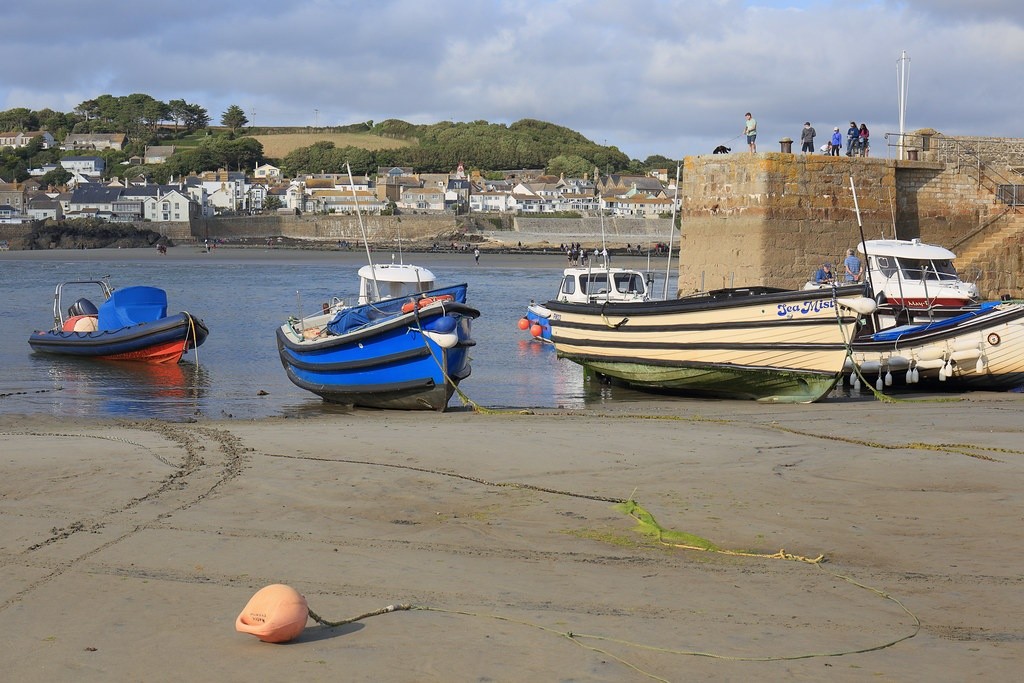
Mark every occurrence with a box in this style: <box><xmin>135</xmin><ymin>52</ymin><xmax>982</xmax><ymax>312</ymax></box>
<box><xmin>401</xmin><ymin>294</ymin><xmax>455</xmax><ymax>314</ymax></box>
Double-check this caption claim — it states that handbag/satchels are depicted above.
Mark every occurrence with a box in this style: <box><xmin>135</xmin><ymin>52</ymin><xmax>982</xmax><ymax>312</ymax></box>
<box><xmin>838</xmin><ymin>143</ymin><xmax>842</xmax><ymax>148</ymax></box>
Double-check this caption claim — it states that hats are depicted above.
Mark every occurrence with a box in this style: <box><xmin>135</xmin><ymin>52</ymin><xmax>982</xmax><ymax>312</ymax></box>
<box><xmin>833</xmin><ymin>127</ymin><xmax>839</xmax><ymax>130</ymax></box>
<box><xmin>824</xmin><ymin>263</ymin><xmax>831</xmax><ymax>270</ymax></box>
<box><xmin>804</xmin><ymin>121</ymin><xmax>810</xmax><ymax>126</ymax></box>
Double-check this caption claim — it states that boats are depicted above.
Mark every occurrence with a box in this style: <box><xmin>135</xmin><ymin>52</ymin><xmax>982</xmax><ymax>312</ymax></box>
<box><xmin>545</xmin><ymin>166</ymin><xmax>877</xmax><ymax>404</ymax></box>
<box><xmin>527</xmin><ymin>206</ymin><xmax>653</xmax><ymax>345</ymax></box>
<box><xmin>276</xmin><ymin>160</ymin><xmax>481</xmax><ymax>414</ymax></box>
<box><xmin>27</xmin><ymin>280</ymin><xmax>209</xmax><ymax>365</ymax></box>
<box><xmin>804</xmin><ymin>176</ymin><xmax>1024</xmax><ymax>387</ymax></box>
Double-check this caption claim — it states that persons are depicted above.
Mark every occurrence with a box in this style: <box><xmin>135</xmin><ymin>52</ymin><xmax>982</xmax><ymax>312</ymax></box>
<box><xmin>843</xmin><ymin>248</ymin><xmax>863</xmax><ymax>284</ymax></box>
<box><xmin>743</xmin><ymin>112</ymin><xmax>758</xmax><ymax>154</ymax></box>
<box><xmin>433</xmin><ymin>240</ymin><xmax>670</xmax><ymax>267</ymax></box>
<box><xmin>800</xmin><ymin>122</ymin><xmax>816</xmax><ymax>155</ymax></box>
<box><xmin>820</xmin><ymin>122</ymin><xmax>870</xmax><ymax>157</ymax></box>
<box><xmin>816</xmin><ymin>263</ymin><xmax>835</xmax><ymax>284</ymax></box>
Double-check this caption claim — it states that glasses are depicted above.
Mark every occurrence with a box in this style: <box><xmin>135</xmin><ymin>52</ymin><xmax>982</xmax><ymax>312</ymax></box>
<box><xmin>850</xmin><ymin>126</ymin><xmax>855</xmax><ymax>127</ymax></box>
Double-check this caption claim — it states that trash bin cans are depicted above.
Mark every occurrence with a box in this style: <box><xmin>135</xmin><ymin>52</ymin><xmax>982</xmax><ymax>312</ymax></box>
<box><xmin>907</xmin><ymin>150</ymin><xmax>919</xmax><ymax>161</ymax></box>
<box><xmin>779</xmin><ymin>139</ymin><xmax>794</xmax><ymax>153</ymax></box>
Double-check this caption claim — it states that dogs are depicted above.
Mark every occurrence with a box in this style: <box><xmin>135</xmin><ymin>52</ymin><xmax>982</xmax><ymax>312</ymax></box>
<box><xmin>713</xmin><ymin>145</ymin><xmax>731</xmax><ymax>154</ymax></box>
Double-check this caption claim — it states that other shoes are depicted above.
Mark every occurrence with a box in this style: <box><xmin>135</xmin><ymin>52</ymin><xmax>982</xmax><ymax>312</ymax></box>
<box><xmin>860</xmin><ymin>153</ymin><xmax>864</xmax><ymax>155</ymax></box>
<box><xmin>854</xmin><ymin>152</ymin><xmax>858</xmax><ymax>154</ymax></box>
<box><xmin>846</xmin><ymin>152</ymin><xmax>852</xmax><ymax>157</ymax></box>
